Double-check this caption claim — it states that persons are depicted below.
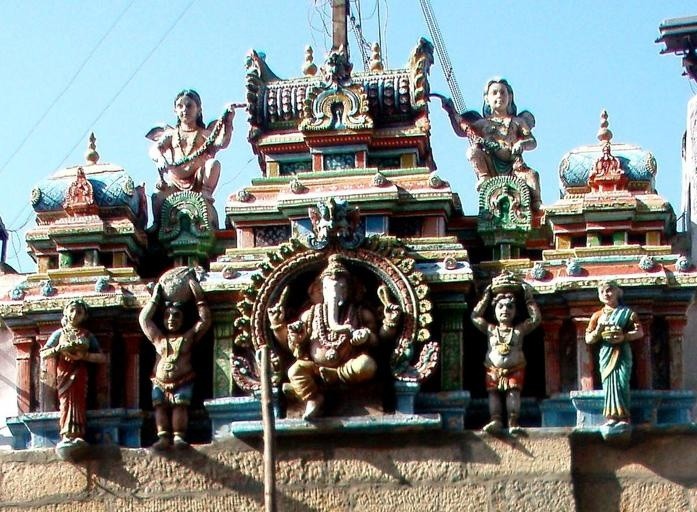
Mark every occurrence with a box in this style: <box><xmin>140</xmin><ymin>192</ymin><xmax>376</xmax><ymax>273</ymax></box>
<box><xmin>445</xmin><ymin>78</ymin><xmax>542</xmax><ymax>219</ymax></box>
<box><xmin>260</xmin><ymin>260</ymin><xmax>401</xmax><ymax>417</ymax></box>
<box><xmin>38</xmin><ymin>299</ymin><xmax>106</xmax><ymax>459</ymax></box>
<box><xmin>468</xmin><ymin>282</ymin><xmax>542</xmax><ymax>434</ymax></box>
<box><xmin>147</xmin><ymin>89</ymin><xmax>235</xmax><ymax>232</ymax></box>
<box><xmin>582</xmin><ymin>278</ymin><xmax>644</xmax><ymax>427</ymax></box>
<box><xmin>138</xmin><ymin>278</ymin><xmax>214</xmax><ymax>447</ymax></box>
